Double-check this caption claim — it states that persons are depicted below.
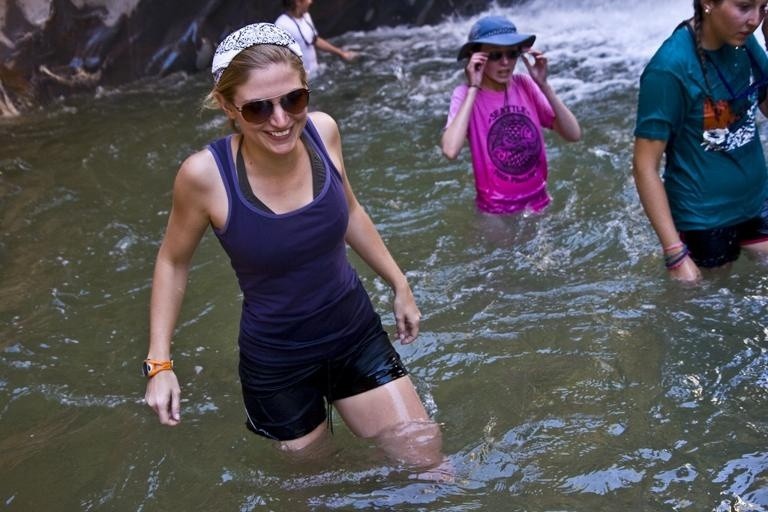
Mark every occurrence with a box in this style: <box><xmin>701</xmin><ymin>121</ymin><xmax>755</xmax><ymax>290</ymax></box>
<box><xmin>440</xmin><ymin>13</ymin><xmax>582</xmax><ymax>224</ymax></box>
<box><xmin>270</xmin><ymin>0</ymin><xmax>358</xmax><ymax>83</ymax></box>
<box><xmin>143</xmin><ymin>21</ymin><xmax>456</xmax><ymax>483</ymax></box>
<box><xmin>630</xmin><ymin>1</ymin><xmax>768</xmax><ymax>282</ymax></box>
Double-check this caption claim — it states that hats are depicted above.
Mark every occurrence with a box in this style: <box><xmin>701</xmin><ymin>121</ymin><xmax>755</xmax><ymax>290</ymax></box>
<box><xmin>457</xmin><ymin>15</ymin><xmax>536</xmax><ymax>61</ymax></box>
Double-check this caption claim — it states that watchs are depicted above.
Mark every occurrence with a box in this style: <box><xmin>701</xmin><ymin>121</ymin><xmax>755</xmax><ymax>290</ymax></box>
<box><xmin>142</xmin><ymin>359</ymin><xmax>174</xmax><ymax>378</ymax></box>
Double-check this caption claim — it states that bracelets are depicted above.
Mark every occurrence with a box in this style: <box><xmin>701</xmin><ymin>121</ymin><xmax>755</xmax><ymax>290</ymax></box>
<box><xmin>662</xmin><ymin>242</ymin><xmax>689</xmax><ymax>271</ymax></box>
<box><xmin>467</xmin><ymin>83</ymin><xmax>482</xmax><ymax>90</ymax></box>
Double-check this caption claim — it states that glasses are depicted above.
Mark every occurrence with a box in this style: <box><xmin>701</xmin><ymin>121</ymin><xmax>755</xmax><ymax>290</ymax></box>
<box><xmin>485</xmin><ymin>49</ymin><xmax>522</xmax><ymax>61</ymax></box>
<box><xmin>227</xmin><ymin>80</ymin><xmax>310</xmax><ymax>123</ymax></box>
